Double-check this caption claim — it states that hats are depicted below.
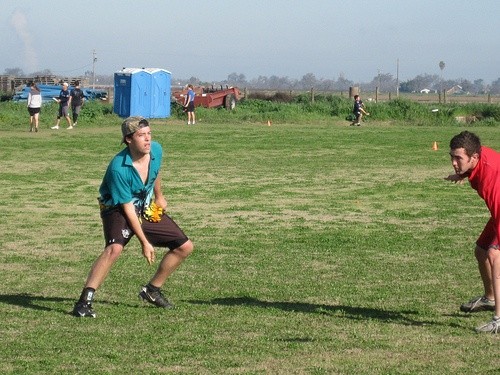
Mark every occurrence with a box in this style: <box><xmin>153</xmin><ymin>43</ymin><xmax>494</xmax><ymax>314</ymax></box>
<box><xmin>119</xmin><ymin>115</ymin><xmax>150</xmax><ymax>146</ymax></box>
<box><xmin>61</xmin><ymin>83</ymin><xmax>68</xmax><ymax>87</ymax></box>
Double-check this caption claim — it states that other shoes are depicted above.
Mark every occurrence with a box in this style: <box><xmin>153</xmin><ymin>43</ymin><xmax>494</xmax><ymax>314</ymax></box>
<box><xmin>35</xmin><ymin>129</ymin><xmax>38</xmax><ymax>132</ymax></box>
<box><xmin>192</xmin><ymin>122</ymin><xmax>195</xmax><ymax>124</ymax></box>
<box><xmin>50</xmin><ymin>126</ymin><xmax>59</xmax><ymax>129</ymax></box>
<box><xmin>350</xmin><ymin>124</ymin><xmax>354</xmax><ymax>126</ymax></box>
<box><xmin>67</xmin><ymin>126</ymin><xmax>72</xmax><ymax>129</ymax></box>
<box><xmin>73</xmin><ymin>121</ymin><xmax>77</xmax><ymax>126</ymax></box>
<box><xmin>187</xmin><ymin>122</ymin><xmax>190</xmax><ymax>125</ymax></box>
<box><xmin>29</xmin><ymin>129</ymin><xmax>33</xmax><ymax>132</ymax></box>
<box><xmin>357</xmin><ymin>124</ymin><xmax>360</xmax><ymax>126</ymax></box>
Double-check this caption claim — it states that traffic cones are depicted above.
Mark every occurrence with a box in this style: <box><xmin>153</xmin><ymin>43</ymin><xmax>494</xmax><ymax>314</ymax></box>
<box><xmin>431</xmin><ymin>140</ymin><xmax>439</xmax><ymax>151</ymax></box>
<box><xmin>267</xmin><ymin>120</ymin><xmax>271</xmax><ymax>127</ymax></box>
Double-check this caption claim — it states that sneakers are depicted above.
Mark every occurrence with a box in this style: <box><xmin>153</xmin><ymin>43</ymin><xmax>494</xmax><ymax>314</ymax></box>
<box><xmin>475</xmin><ymin>315</ymin><xmax>500</xmax><ymax>334</ymax></box>
<box><xmin>460</xmin><ymin>295</ymin><xmax>496</xmax><ymax>311</ymax></box>
<box><xmin>73</xmin><ymin>300</ymin><xmax>96</xmax><ymax>318</ymax></box>
<box><xmin>137</xmin><ymin>284</ymin><xmax>172</xmax><ymax>310</ymax></box>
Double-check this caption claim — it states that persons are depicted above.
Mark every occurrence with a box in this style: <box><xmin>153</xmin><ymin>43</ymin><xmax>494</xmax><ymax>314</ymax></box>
<box><xmin>444</xmin><ymin>131</ymin><xmax>500</xmax><ymax>332</ymax></box>
<box><xmin>68</xmin><ymin>81</ymin><xmax>85</xmax><ymax>126</ymax></box>
<box><xmin>50</xmin><ymin>82</ymin><xmax>73</xmax><ymax>130</ymax></box>
<box><xmin>349</xmin><ymin>94</ymin><xmax>370</xmax><ymax>127</ymax></box>
<box><xmin>71</xmin><ymin>116</ymin><xmax>193</xmax><ymax>319</ymax></box>
<box><xmin>182</xmin><ymin>84</ymin><xmax>195</xmax><ymax>126</ymax></box>
<box><xmin>27</xmin><ymin>81</ymin><xmax>42</xmax><ymax>132</ymax></box>
